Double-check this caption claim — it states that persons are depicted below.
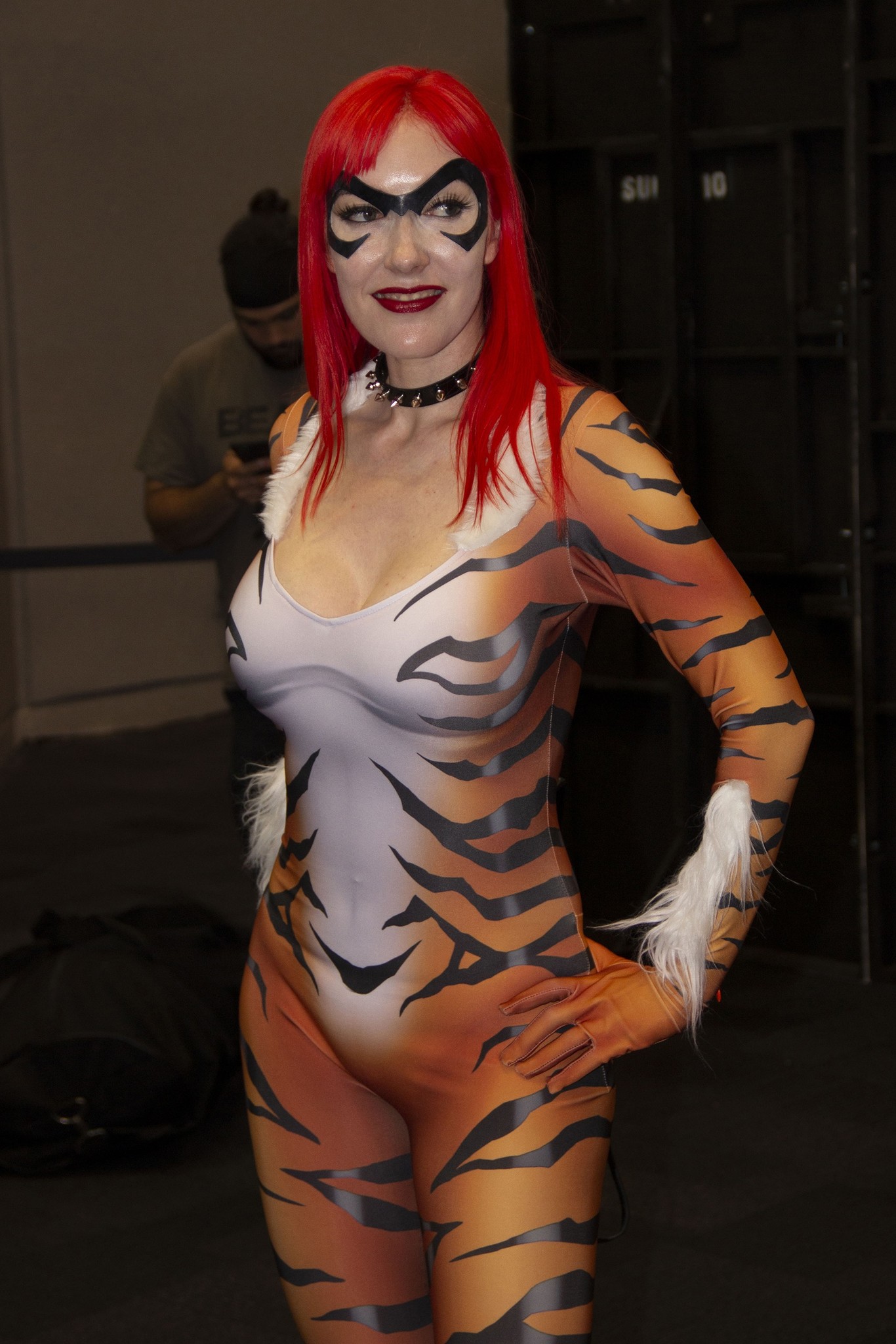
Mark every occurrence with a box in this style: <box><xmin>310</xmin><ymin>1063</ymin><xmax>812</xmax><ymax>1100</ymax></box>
<box><xmin>224</xmin><ymin>66</ymin><xmax>816</xmax><ymax>1344</ymax></box>
<box><xmin>132</xmin><ymin>186</ymin><xmax>361</xmax><ymax>830</ymax></box>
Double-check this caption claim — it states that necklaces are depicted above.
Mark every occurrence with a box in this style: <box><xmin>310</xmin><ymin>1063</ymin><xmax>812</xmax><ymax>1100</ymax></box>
<box><xmin>364</xmin><ymin>350</ymin><xmax>482</xmax><ymax>411</ymax></box>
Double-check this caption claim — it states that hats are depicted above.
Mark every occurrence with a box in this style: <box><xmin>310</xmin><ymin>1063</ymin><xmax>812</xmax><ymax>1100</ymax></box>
<box><xmin>219</xmin><ymin>212</ymin><xmax>299</xmax><ymax>309</ymax></box>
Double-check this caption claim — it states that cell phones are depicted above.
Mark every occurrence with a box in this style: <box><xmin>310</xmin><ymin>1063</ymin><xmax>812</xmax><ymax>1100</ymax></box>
<box><xmin>232</xmin><ymin>439</ymin><xmax>273</xmax><ymax>478</ymax></box>
